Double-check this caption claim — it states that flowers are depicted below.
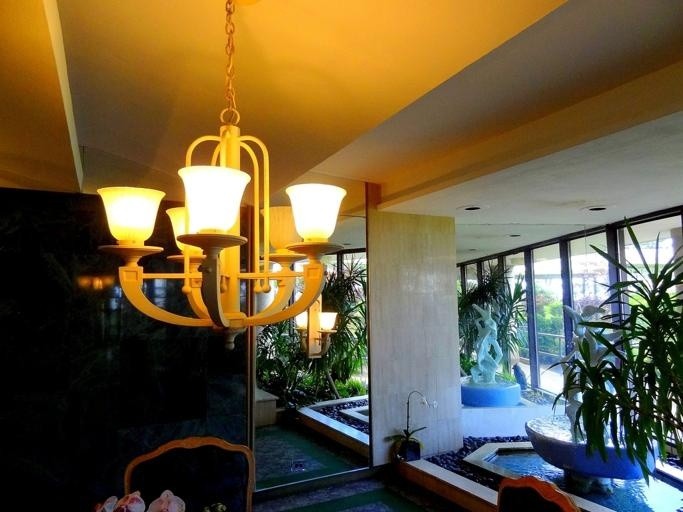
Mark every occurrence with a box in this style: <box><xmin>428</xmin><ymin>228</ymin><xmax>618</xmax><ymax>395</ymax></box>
<box><xmin>384</xmin><ymin>390</ymin><xmax>437</xmax><ymax>458</ymax></box>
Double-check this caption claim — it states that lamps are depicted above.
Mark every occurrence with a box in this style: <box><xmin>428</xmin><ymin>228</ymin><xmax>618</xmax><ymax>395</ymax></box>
<box><xmin>94</xmin><ymin>2</ymin><xmax>346</xmax><ymax>348</ymax></box>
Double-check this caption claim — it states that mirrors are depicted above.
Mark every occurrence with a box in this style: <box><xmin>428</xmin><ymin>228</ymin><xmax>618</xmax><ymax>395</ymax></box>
<box><xmin>256</xmin><ymin>163</ymin><xmax>372</xmax><ymax>495</ymax></box>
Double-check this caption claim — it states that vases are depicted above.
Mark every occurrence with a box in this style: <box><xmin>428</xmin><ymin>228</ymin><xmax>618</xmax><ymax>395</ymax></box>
<box><xmin>399</xmin><ymin>443</ymin><xmax>420</xmax><ymax>461</ymax></box>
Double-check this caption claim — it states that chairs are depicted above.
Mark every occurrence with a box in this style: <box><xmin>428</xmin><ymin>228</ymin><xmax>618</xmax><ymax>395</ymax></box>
<box><xmin>124</xmin><ymin>437</ymin><xmax>256</xmax><ymax>512</ymax></box>
<box><xmin>498</xmin><ymin>474</ymin><xmax>581</xmax><ymax>512</ymax></box>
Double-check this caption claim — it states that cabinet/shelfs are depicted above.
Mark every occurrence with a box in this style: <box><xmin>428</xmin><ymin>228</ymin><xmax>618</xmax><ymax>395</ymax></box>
<box><xmin>2</xmin><ymin>187</ymin><xmax>255</xmax><ymax>509</ymax></box>
